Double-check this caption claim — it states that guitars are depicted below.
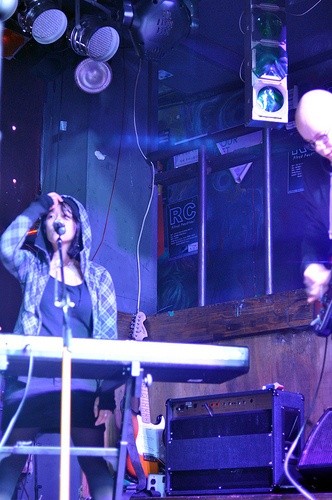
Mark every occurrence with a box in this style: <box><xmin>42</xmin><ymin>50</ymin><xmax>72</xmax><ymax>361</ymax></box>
<box><xmin>125</xmin><ymin>312</ymin><xmax>166</xmax><ymax>479</ymax></box>
<box><xmin>312</xmin><ymin>266</ymin><xmax>331</xmax><ymax>340</ymax></box>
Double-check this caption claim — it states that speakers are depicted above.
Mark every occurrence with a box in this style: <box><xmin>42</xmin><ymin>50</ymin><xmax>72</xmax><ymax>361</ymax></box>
<box><xmin>299</xmin><ymin>408</ymin><xmax>332</xmax><ymax>493</ymax></box>
<box><xmin>166</xmin><ymin>389</ymin><xmax>304</xmax><ymax>493</ymax></box>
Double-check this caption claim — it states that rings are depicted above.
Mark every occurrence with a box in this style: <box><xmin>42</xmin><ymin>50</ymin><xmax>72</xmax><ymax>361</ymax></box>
<box><xmin>104</xmin><ymin>412</ymin><xmax>107</xmax><ymax>417</ymax></box>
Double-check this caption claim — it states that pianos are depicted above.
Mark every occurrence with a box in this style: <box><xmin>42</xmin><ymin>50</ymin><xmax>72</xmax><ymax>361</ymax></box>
<box><xmin>0</xmin><ymin>332</ymin><xmax>250</xmax><ymax>500</ymax></box>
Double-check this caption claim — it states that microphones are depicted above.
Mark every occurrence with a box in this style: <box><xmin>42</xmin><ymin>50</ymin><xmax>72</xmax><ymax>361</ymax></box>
<box><xmin>53</xmin><ymin>220</ymin><xmax>66</xmax><ymax>235</ymax></box>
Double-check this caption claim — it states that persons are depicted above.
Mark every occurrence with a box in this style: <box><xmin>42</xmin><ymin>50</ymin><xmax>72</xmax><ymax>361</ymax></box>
<box><xmin>295</xmin><ymin>88</ymin><xmax>332</xmax><ymax>303</ymax></box>
<box><xmin>0</xmin><ymin>191</ymin><xmax>120</xmax><ymax>500</ymax></box>
